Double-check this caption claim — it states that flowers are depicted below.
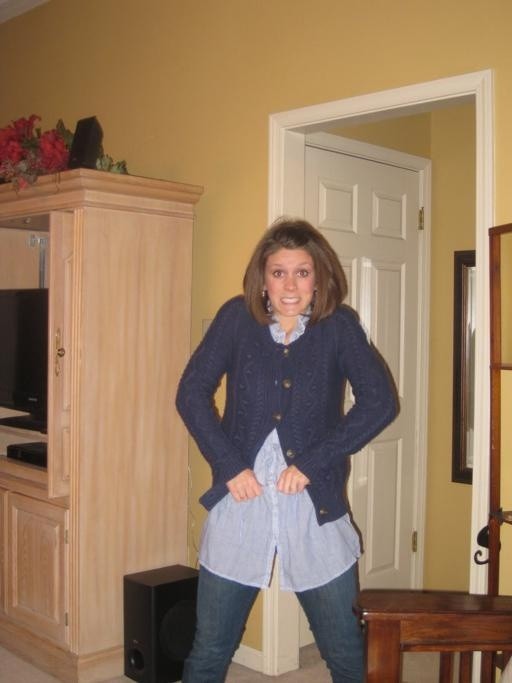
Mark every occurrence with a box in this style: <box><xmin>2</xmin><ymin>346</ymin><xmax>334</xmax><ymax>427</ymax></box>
<box><xmin>1</xmin><ymin>111</ymin><xmax>129</xmax><ymax>192</ymax></box>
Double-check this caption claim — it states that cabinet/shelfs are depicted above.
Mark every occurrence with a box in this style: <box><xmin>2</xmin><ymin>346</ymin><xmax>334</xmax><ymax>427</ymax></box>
<box><xmin>0</xmin><ymin>163</ymin><xmax>207</xmax><ymax>682</ymax></box>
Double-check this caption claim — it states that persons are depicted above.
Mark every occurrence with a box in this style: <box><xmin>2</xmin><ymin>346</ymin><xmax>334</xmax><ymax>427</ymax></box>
<box><xmin>174</xmin><ymin>218</ymin><xmax>401</xmax><ymax>680</ymax></box>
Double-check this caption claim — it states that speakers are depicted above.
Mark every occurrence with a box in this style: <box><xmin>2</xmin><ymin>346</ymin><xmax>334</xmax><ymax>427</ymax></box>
<box><xmin>123</xmin><ymin>563</ymin><xmax>198</xmax><ymax>680</ymax></box>
<box><xmin>69</xmin><ymin>116</ymin><xmax>104</xmax><ymax>170</ymax></box>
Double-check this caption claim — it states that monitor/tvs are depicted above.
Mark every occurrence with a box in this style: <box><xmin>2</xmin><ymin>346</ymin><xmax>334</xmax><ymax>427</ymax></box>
<box><xmin>0</xmin><ymin>288</ymin><xmax>46</xmax><ymax>432</ymax></box>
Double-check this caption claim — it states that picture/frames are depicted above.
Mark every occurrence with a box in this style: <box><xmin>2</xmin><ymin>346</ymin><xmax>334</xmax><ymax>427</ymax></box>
<box><xmin>450</xmin><ymin>247</ymin><xmax>477</xmax><ymax>487</ymax></box>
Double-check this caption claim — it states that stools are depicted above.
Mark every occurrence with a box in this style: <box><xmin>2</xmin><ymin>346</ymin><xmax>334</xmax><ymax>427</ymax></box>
<box><xmin>349</xmin><ymin>587</ymin><xmax>511</xmax><ymax>681</ymax></box>
